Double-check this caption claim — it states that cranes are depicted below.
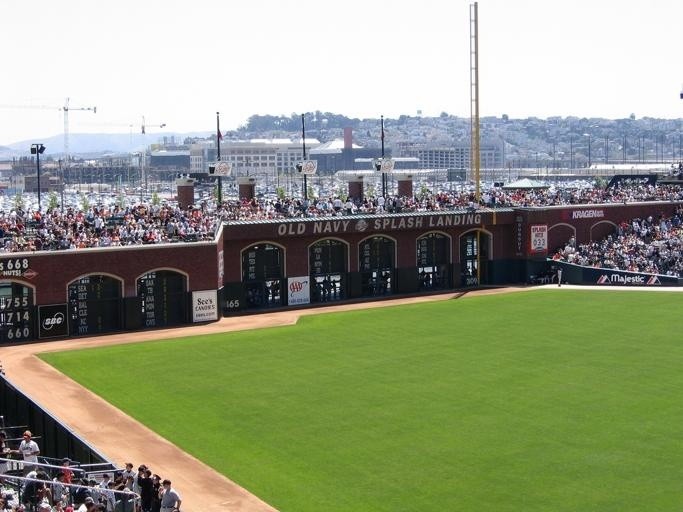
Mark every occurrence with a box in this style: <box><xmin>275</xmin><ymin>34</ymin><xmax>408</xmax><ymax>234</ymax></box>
<box><xmin>58</xmin><ymin>97</ymin><xmax>97</xmax><ymax>156</ymax></box>
<box><xmin>128</xmin><ymin>113</ymin><xmax>165</xmax><ymax>134</ymax></box>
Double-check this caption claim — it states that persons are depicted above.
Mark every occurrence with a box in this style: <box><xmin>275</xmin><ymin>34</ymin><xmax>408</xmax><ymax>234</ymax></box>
<box><xmin>0</xmin><ymin>427</ymin><xmax>181</xmax><ymax>512</ymax></box>
<box><xmin>0</xmin><ymin>177</ymin><xmax>683</xmax><ymax>283</ymax></box>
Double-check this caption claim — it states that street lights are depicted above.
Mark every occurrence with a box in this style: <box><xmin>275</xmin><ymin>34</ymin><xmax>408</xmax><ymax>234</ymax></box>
<box><xmin>30</xmin><ymin>141</ymin><xmax>46</xmax><ymax>206</ymax></box>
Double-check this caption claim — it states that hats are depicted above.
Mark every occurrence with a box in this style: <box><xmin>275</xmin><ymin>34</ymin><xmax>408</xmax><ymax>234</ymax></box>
<box><xmin>23</xmin><ymin>430</ymin><xmax>32</xmax><ymax>439</ymax></box>
<box><xmin>161</xmin><ymin>479</ymin><xmax>171</xmax><ymax>484</ymax></box>
<box><xmin>125</xmin><ymin>463</ymin><xmax>133</xmax><ymax>468</ymax></box>
<box><xmin>80</xmin><ymin>478</ymin><xmax>89</xmax><ymax>485</ymax></box>
<box><xmin>138</xmin><ymin>464</ymin><xmax>151</xmax><ymax>475</ymax></box>
<box><xmin>61</xmin><ymin>457</ymin><xmax>72</xmax><ymax>463</ymax></box>
<box><xmin>108</xmin><ymin>481</ymin><xmax>116</xmax><ymax>486</ymax></box>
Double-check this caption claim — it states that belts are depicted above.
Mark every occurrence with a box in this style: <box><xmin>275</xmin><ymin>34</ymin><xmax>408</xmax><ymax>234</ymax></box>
<box><xmin>162</xmin><ymin>505</ymin><xmax>174</xmax><ymax>508</ymax></box>
